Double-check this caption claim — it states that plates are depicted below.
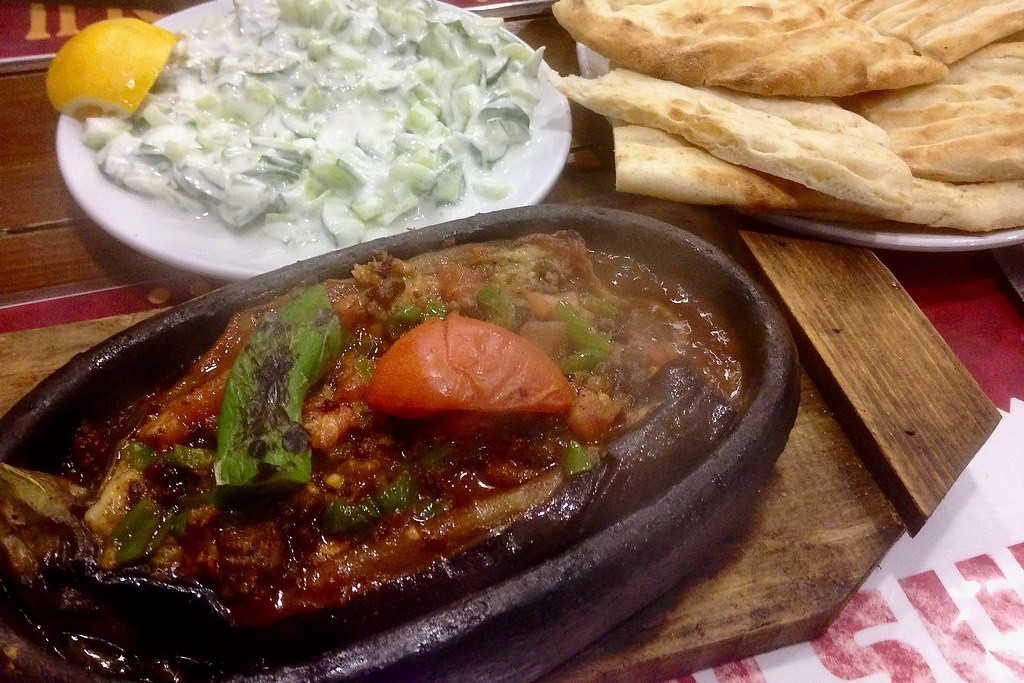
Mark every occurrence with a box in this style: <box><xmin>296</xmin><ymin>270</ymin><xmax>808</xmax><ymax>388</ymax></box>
<box><xmin>0</xmin><ymin>204</ymin><xmax>803</xmax><ymax>683</ymax></box>
<box><xmin>56</xmin><ymin>0</ymin><xmax>574</xmax><ymax>281</ymax></box>
<box><xmin>575</xmin><ymin>39</ymin><xmax>1024</xmax><ymax>253</ymax></box>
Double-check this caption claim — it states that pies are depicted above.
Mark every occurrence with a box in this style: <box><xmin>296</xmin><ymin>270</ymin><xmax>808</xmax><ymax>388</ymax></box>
<box><xmin>548</xmin><ymin>0</ymin><xmax>1024</xmax><ymax>232</ymax></box>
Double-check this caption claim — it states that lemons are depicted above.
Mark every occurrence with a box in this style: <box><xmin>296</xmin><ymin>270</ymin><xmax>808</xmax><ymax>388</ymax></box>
<box><xmin>43</xmin><ymin>20</ymin><xmax>175</xmax><ymax>117</ymax></box>
